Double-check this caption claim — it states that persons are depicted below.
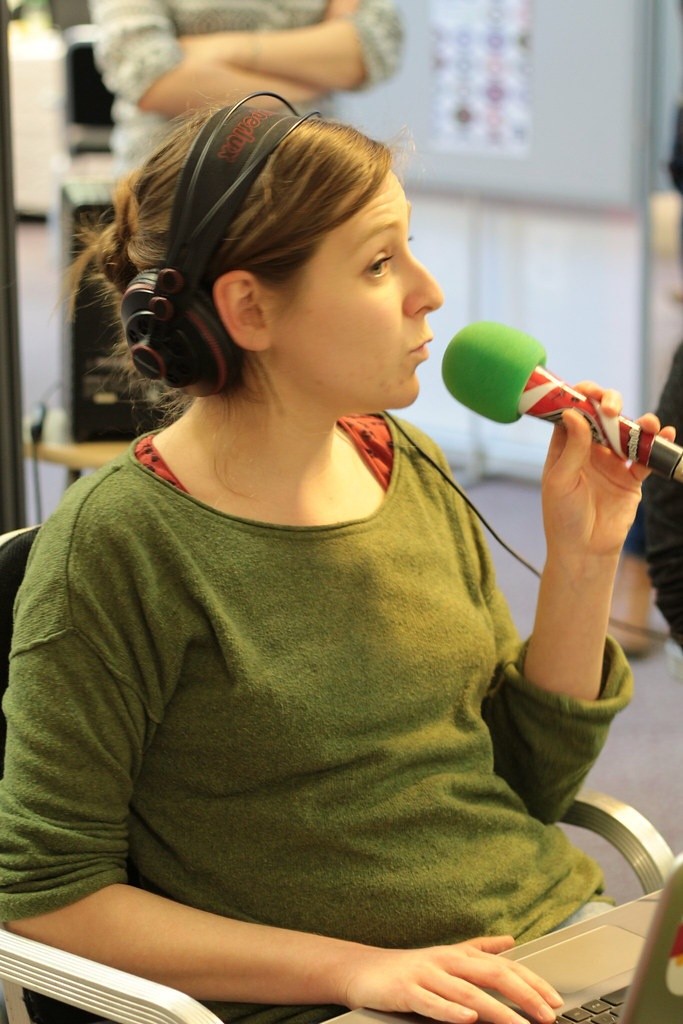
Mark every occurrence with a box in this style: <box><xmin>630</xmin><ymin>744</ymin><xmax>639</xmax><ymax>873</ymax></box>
<box><xmin>76</xmin><ymin>0</ymin><xmax>414</xmax><ymax>173</ymax></box>
<box><xmin>607</xmin><ymin>83</ymin><xmax>683</xmax><ymax>665</ymax></box>
<box><xmin>0</xmin><ymin>98</ymin><xmax>681</xmax><ymax>1020</ymax></box>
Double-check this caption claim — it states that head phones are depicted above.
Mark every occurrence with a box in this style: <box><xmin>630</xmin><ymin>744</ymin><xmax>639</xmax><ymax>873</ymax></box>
<box><xmin>121</xmin><ymin>91</ymin><xmax>322</xmax><ymax>397</ymax></box>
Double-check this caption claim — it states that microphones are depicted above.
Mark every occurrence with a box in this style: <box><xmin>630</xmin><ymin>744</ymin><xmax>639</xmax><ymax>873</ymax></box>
<box><xmin>441</xmin><ymin>320</ymin><xmax>683</xmax><ymax>483</ymax></box>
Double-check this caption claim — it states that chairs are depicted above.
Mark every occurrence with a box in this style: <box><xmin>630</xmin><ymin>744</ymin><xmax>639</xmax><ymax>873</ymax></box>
<box><xmin>0</xmin><ymin>526</ymin><xmax>675</xmax><ymax>1023</ymax></box>
